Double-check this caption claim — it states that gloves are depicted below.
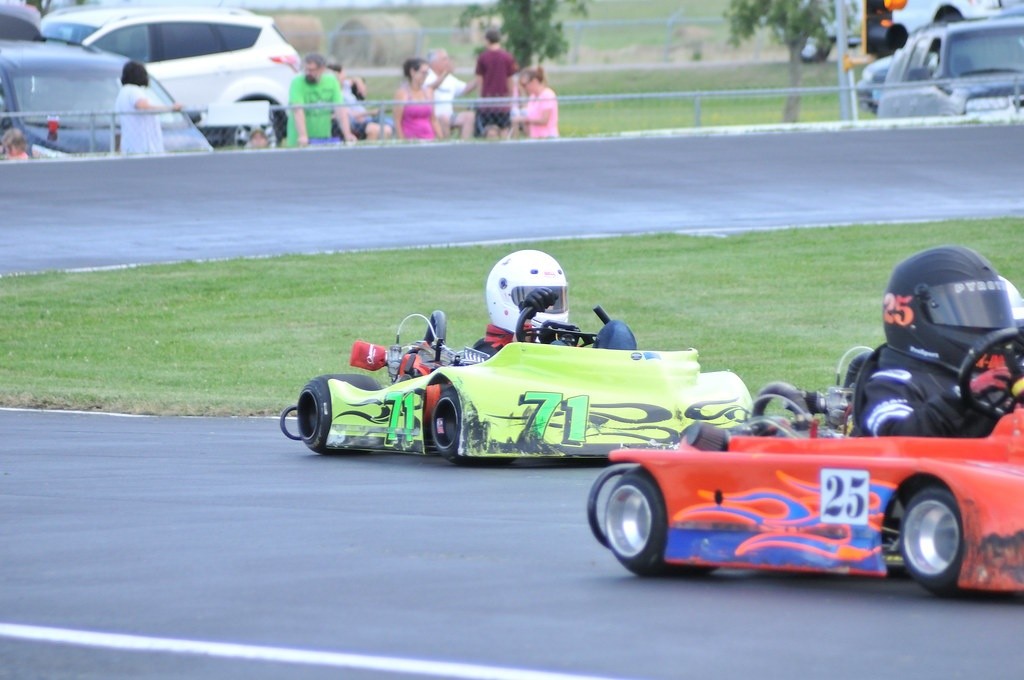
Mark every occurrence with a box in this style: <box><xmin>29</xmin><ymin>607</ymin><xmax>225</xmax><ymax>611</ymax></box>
<box><xmin>523</xmin><ymin>287</ymin><xmax>555</xmax><ymax>319</ymax></box>
<box><xmin>959</xmin><ymin>365</ymin><xmax>1016</xmax><ymax>418</ymax></box>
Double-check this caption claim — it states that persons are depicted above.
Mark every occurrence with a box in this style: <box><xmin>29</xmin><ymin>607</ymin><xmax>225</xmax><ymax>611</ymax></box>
<box><xmin>244</xmin><ymin>28</ymin><xmax>560</xmax><ymax>148</ymax></box>
<box><xmin>0</xmin><ymin>127</ymin><xmax>32</xmax><ymax>159</ymax></box>
<box><xmin>117</xmin><ymin>61</ymin><xmax>182</xmax><ymax>158</ymax></box>
<box><xmin>854</xmin><ymin>243</ymin><xmax>1024</xmax><ymax>438</ymax></box>
<box><xmin>471</xmin><ymin>249</ymin><xmax>570</xmax><ymax>357</ymax></box>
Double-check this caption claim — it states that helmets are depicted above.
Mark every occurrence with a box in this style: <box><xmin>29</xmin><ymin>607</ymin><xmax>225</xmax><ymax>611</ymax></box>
<box><xmin>487</xmin><ymin>249</ymin><xmax>569</xmax><ymax>333</ymax></box>
<box><xmin>882</xmin><ymin>246</ymin><xmax>1011</xmax><ymax>369</ymax></box>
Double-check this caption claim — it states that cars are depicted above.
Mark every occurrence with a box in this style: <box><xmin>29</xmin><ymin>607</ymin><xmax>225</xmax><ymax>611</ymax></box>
<box><xmin>852</xmin><ymin>0</ymin><xmax>1024</xmax><ymax>123</ymax></box>
<box><xmin>0</xmin><ymin>1</ymin><xmax>312</xmax><ymax>157</ymax></box>
